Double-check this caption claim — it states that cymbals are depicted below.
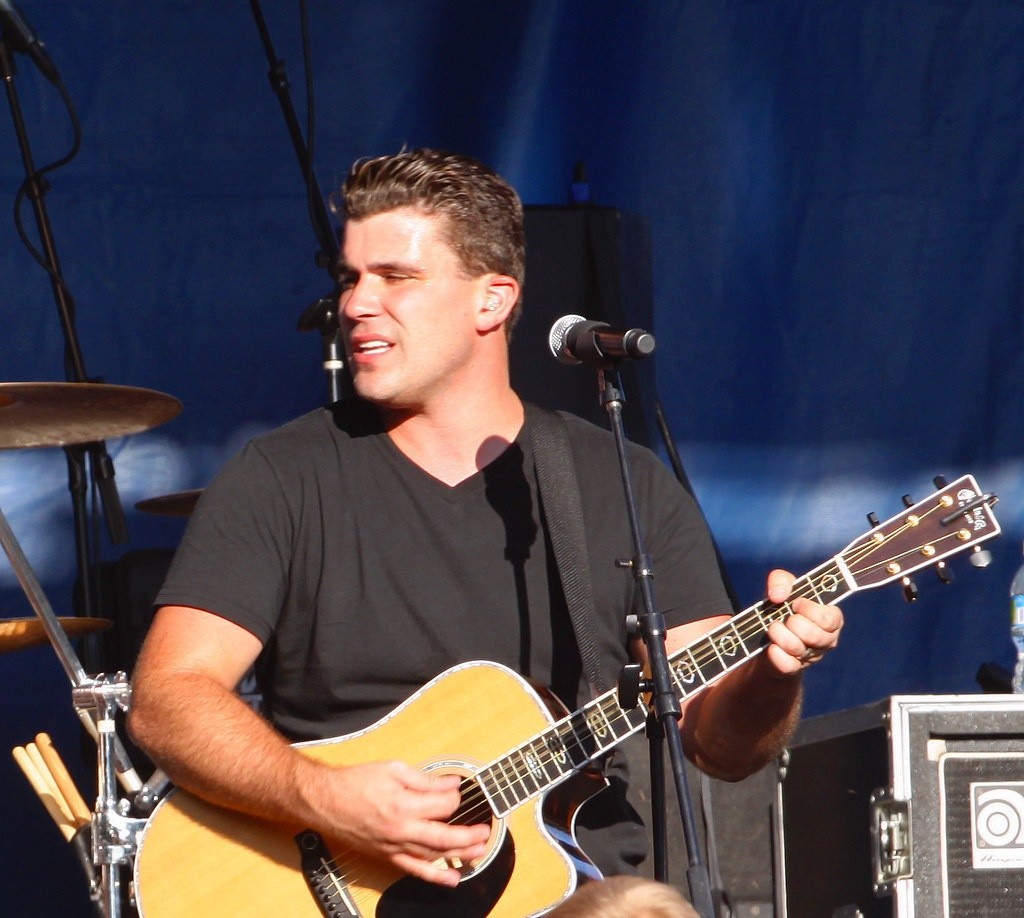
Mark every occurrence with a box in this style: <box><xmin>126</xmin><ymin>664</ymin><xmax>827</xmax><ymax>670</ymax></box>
<box><xmin>133</xmin><ymin>487</ymin><xmax>205</xmax><ymax>517</ymax></box>
<box><xmin>0</xmin><ymin>615</ymin><xmax>115</xmax><ymax>648</ymax></box>
<box><xmin>0</xmin><ymin>380</ymin><xmax>181</xmax><ymax>454</ymax></box>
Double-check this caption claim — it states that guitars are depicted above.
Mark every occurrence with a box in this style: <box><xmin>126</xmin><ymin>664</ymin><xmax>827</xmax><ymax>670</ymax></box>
<box><xmin>132</xmin><ymin>470</ymin><xmax>1003</xmax><ymax>918</ymax></box>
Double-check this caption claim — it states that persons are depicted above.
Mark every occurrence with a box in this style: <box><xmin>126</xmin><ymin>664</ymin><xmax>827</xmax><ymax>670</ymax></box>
<box><xmin>543</xmin><ymin>874</ymin><xmax>701</xmax><ymax>918</ymax></box>
<box><xmin>125</xmin><ymin>146</ymin><xmax>844</xmax><ymax>918</ymax></box>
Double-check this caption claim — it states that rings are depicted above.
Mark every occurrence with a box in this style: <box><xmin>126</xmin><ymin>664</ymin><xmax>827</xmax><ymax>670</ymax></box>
<box><xmin>796</xmin><ymin>647</ymin><xmax>813</xmax><ymax>661</ymax></box>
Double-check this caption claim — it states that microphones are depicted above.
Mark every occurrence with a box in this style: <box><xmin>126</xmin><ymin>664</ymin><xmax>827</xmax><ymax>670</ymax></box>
<box><xmin>549</xmin><ymin>314</ymin><xmax>656</xmax><ymax>365</ymax></box>
